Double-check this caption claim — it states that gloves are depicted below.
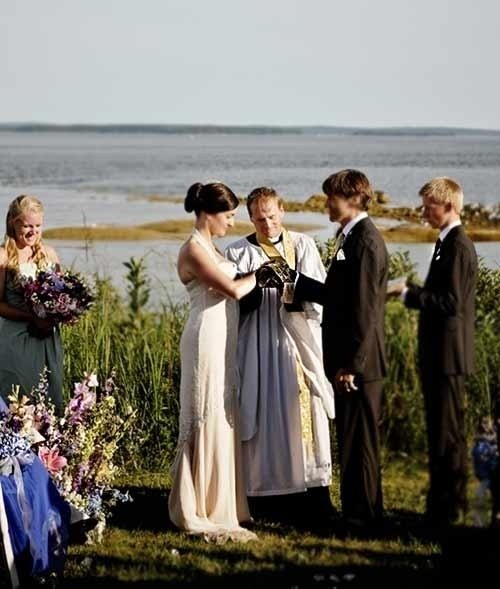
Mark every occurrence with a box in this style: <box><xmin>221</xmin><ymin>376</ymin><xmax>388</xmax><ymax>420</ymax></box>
<box><xmin>255</xmin><ymin>260</ymin><xmax>297</xmax><ymax>288</ymax></box>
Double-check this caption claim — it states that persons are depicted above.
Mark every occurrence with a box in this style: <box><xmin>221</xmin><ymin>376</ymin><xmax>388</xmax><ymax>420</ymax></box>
<box><xmin>168</xmin><ymin>183</ymin><xmax>275</xmax><ymax>545</ymax></box>
<box><xmin>387</xmin><ymin>176</ymin><xmax>480</xmax><ymax>522</ymax></box>
<box><xmin>284</xmin><ymin>169</ymin><xmax>388</xmax><ymax>533</ymax></box>
<box><xmin>0</xmin><ymin>194</ymin><xmax>64</xmax><ymax>415</ymax></box>
<box><xmin>225</xmin><ymin>186</ymin><xmax>336</xmax><ymax>515</ymax></box>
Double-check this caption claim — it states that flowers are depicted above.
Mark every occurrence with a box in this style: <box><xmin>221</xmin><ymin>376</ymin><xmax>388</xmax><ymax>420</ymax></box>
<box><xmin>1</xmin><ymin>360</ymin><xmax>137</xmax><ymax>546</ymax></box>
<box><xmin>14</xmin><ymin>262</ymin><xmax>101</xmax><ymax>339</ymax></box>
